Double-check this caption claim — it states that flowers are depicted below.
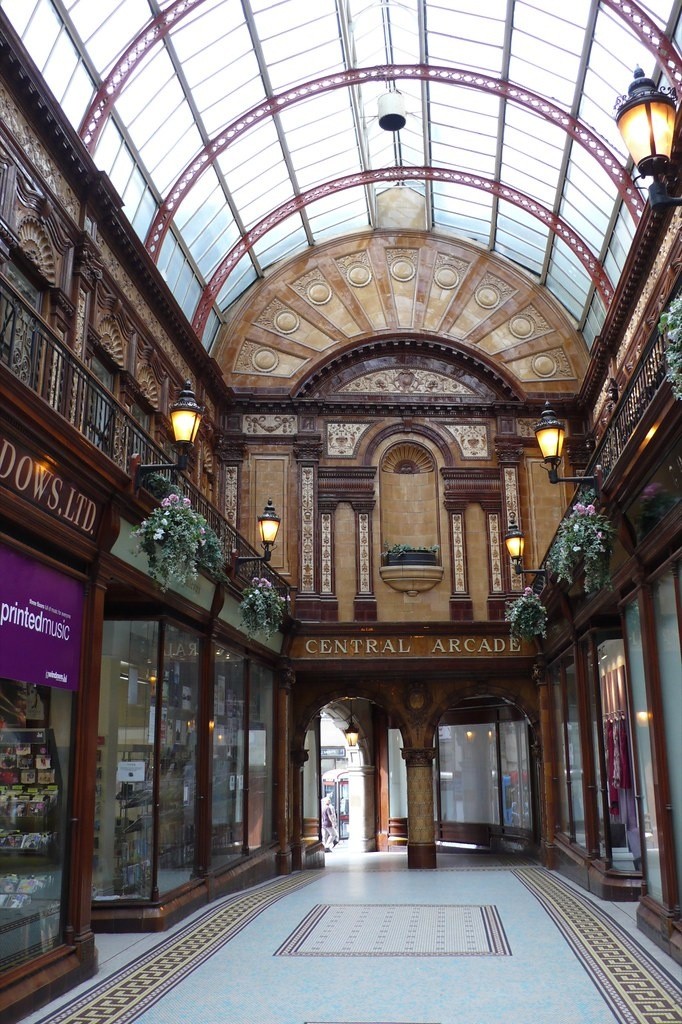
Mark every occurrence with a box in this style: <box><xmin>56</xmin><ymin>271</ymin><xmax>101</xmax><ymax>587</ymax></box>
<box><xmin>504</xmin><ymin>587</ymin><xmax>548</xmax><ymax>644</ymax></box>
<box><xmin>128</xmin><ymin>493</ymin><xmax>227</xmax><ymax>594</ymax></box>
<box><xmin>237</xmin><ymin>577</ymin><xmax>292</xmax><ymax>641</ymax></box>
<box><xmin>545</xmin><ymin>501</ymin><xmax>615</xmax><ymax>594</ymax></box>
<box><xmin>635</xmin><ymin>483</ymin><xmax>674</xmax><ymax>538</ymax></box>
<box><xmin>657</xmin><ymin>293</ymin><xmax>682</xmax><ymax>400</ymax></box>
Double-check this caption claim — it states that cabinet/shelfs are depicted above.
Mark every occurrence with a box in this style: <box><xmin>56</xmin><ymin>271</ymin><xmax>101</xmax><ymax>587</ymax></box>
<box><xmin>114</xmin><ymin>782</ymin><xmax>154</xmax><ymax>894</ymax></box>
<box><xmin>0</xmin><ymin>725</ymin><xmax>65</xmax><ymax>926</ymax></box>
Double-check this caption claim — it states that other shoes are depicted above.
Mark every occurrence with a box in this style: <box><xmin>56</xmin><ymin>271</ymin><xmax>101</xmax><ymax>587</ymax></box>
<box><xmin>333</xmin><ymin>841</ymin><xmax>338</xmax><ymax>848</ymax></box>
<box><xmin>325</xmin><ymin>848</ymin><xmax>333</xmax><ymax>853</ymax></box>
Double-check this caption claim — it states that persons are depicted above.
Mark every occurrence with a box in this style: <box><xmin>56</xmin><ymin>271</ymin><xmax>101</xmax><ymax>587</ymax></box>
<box><xmin>323</xmin><ymin>797</ymin><xmax>339</xmax><ymax>848</ymax></box>
<box><xmin>26</xmin><ymin>683</ymin><xmax>43</xmax><ymax>720</ymax></box>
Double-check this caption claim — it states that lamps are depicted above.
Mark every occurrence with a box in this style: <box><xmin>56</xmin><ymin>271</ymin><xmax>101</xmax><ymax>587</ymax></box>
<box><xmin>614</xmin><ymin>64</ymin><xmax>682</xmax><ymax>212</ymax></box>
<box><xmin>534</xmin><ymin>399</ymin><xmax>599</xmax><ymax>498</ymax></box>
<box><xmin>235</xmin><ymin>498</ymin><xmax>281</xmax><ymax>576</ymax></box>
<box><xmin>134</xmin><ymin>378</ymin><xmax>206</xmax><ymax>494</ymax></box>
<box><xmin>344</xmin><ymin>700</ymin><xmax>360</xmax><ymax>747</ymax></box>
<box><xmin>504</xmin><ymin>516</ymin><xmax>548</xmax><ymax>585</ymax></box>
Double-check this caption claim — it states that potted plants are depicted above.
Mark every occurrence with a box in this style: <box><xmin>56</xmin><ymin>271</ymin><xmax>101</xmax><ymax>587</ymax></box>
<box><xmin>379</xmin><ymin>540</ymin><xmax>440</xmax><ymax>566</ymax></box>
